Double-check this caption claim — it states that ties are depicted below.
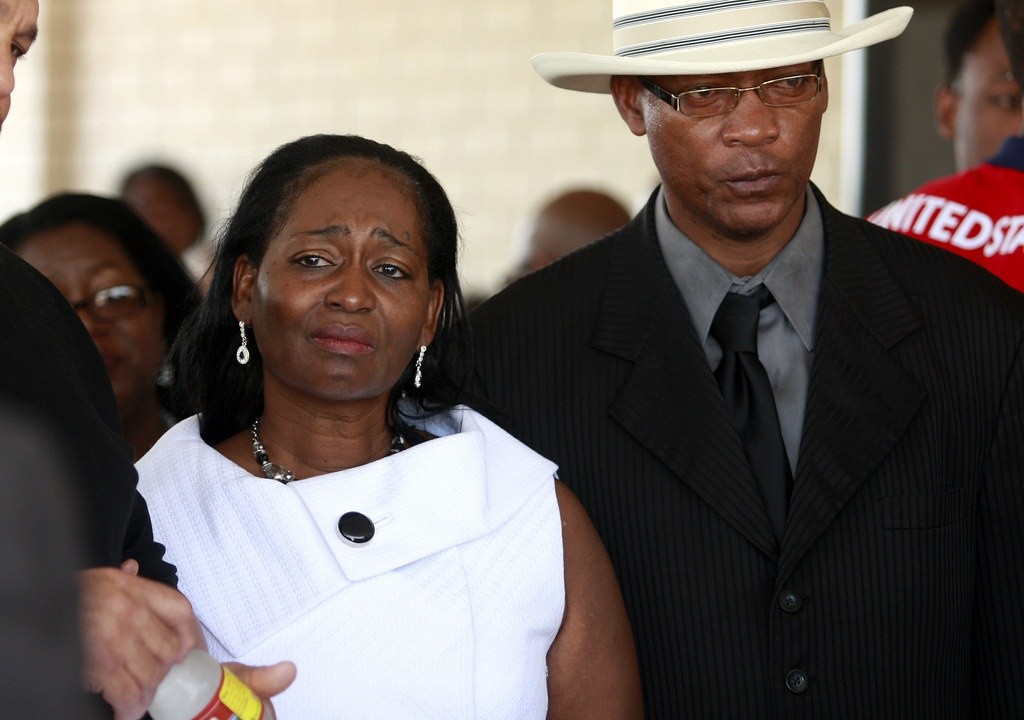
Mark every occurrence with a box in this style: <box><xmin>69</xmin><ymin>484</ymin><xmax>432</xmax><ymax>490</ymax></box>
<box><xmin>710</xmin><ymin>288</ymin><xmax>794</xmax><ymax>551</ymax></box>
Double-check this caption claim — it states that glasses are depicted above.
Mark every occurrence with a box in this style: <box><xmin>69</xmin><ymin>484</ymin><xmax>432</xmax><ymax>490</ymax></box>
<box><xmin>636</xmin><ymin>62</ymin><xmax>824</xmax><ymax>118</ymax></box>
<box><xmin>66</xmin><ymin>284</ymin><xmax>145</xmax><ymax>321</ymax></box>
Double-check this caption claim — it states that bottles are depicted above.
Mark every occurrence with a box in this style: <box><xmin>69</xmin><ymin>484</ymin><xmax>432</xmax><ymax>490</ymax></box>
<box><xmin>146</xmin><ymin>647</ymin><xmax>272</xmax><ymax>719</ymax></box>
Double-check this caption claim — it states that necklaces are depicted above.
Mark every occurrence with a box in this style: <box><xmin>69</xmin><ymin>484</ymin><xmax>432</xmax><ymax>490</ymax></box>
<box><xmin>250</xmin><ymin>413</ymin><xmax>408</xmax><ymax>549</ymax></box>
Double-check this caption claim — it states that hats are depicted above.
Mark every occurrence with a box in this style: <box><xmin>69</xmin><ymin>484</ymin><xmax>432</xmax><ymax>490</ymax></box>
<box><xmin>530</xmin><ymin>0</ymin><xmax>911</xmax><ymax>93</ymax></box>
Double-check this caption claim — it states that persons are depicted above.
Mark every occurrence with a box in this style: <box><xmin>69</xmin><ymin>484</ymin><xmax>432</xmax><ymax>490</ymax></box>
<box><xmin>938</xmin><ymin>0</ymin><xmax>1023</xmax><ymax>174</ymax></box>
<box><xmin>113</xmin><ymin>160</ymin><xmax>223</xmax><ymax>303</ymax></box>
<box><xmin>859</xmin><ymin>1</ymin><xmax>1023</xmax><ymax>287</ymax></box>
<box><xmin>517</xmin><ymin>189</ymin><xmax>632</xmax><ymax>267</ymax></box>
<box><xmin>406</xmin><ymin>1</ymin><xmax>1024</xmax><ymax>720</ymax></box>
<box><xmin>3</xmin><ymin>2</ymin><xmax>298</xmax><ymax>720</ymax></box>
<box><xmin>0</xmin><ymin>188</ymin><xmax>214</xmax><ymax>449</ymax></box>
<box><xmin>119</xmin><ymin>134</ymin><xmax>644</xmax><ymax>720</ymax></box>
